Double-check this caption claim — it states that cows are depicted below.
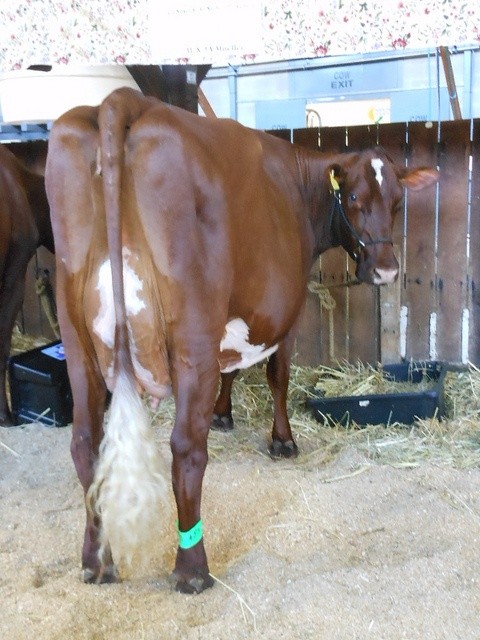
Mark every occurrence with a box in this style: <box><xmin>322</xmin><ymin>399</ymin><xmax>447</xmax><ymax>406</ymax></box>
<box><xmin>44</xmin><ymin>87</ymin><xmax>440</xmax><ymax>595</ymax></box>
<box><xmin>0</xmin><ymin>140</ymin><xmax>58</xmax><ymax>426</ymax></box>
